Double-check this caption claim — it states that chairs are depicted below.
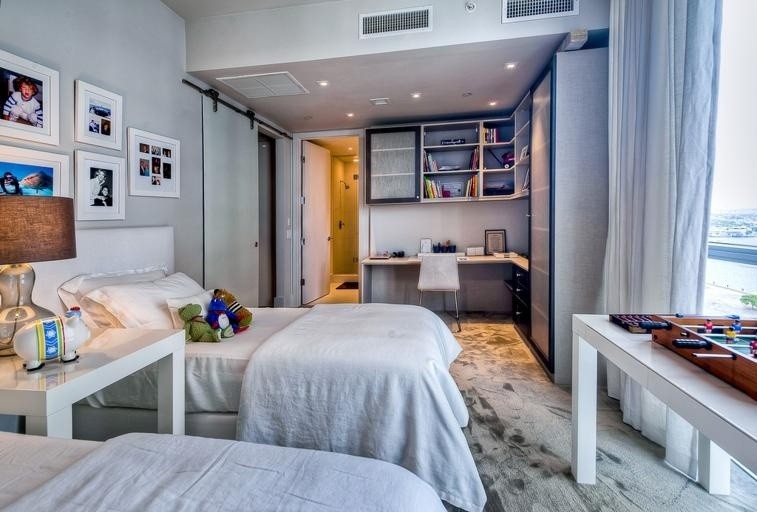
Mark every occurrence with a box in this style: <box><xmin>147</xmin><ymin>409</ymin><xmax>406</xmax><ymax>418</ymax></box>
<box><xmin>417</xmin><ymin>253</ymin><xmax>461</xmax><ymax>332</ymax></box>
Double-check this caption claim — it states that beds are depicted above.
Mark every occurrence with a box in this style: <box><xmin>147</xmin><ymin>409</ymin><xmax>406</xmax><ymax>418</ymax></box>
<box><xmin>31</xmin><ymin>227</ymin><xmax>488</xmax><ymax>512</ymax></box>
<box><xmin>0</xmin><ymin>431</ymin><xmax>447</xmax><ymax>512</ymax></box>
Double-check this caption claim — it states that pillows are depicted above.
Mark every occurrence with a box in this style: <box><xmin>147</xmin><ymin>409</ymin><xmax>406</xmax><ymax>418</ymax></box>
<box><xmin>57</xmin><ymin>265</ymin><xmax>214</xmax><ymax>342</ymax></box>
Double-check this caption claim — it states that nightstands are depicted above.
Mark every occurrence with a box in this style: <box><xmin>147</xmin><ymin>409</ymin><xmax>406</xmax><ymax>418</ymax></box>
<box><xmin>0</xmin><ymin>328</ymin><xmax>185</xmax><ymax>438</ymax></box>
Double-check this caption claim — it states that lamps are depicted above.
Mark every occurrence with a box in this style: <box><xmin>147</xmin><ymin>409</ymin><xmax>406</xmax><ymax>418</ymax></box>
<box><xmin>0</xmin><ymin>196</ymin><xmax>76</xmax><ymax>357</ymax></box>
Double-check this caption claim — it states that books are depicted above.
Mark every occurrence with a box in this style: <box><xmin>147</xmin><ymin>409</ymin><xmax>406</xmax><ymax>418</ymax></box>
<box><xmin>423</xmin><ymin>152</ymin><xmax>443</xmax><ymax>198</ymax></box>
<box><xmin>483</xmin><ymin>127</ymin><xmax>497</xmax><ymax>144</ymax></box>
<box><xmin>463</xmin><ymin>145</ymin><xmax>479</xmax><ymax>197</ymax></box>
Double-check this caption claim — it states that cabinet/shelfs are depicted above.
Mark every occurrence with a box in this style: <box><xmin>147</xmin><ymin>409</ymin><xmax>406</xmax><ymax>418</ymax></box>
<box><xmin>504</xmin><ymin>265</ymin><xmax>529</xmax><ymax>340</ymax></box>
<box><xmin>366</xmin><ymin>89</ymin><xmax>530</xmax><ymax>206</ymax></box>
<box><xmin>529</xmin><ymin>49</ymin><xmax>609</xmax><ymax>385</ymax></box>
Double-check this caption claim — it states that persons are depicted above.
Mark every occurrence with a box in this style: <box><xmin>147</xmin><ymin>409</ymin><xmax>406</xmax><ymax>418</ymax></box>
<box><xmin>89</xmin><ymin>168</ymin><xmax>108</xmax><ymax>206</ymax></box>
<box><xmin>89</xmin><ymin>106</ymin><xmax>111</xmax><ymax>135</ymax></box>
<box><xmin>140</xmin><ymin>145</ymin><xmax>170</xmax><ymax>185</ymax></box>
<box><xmin>0</xmin><ymin>172</ymin><xmax>23</xmax><ymax>196</ymax></box>
<box><xmin>2</xmin><ymin>75</ymin><xmax>43</xmax><ymax>128</ymax></box>
<box><xmin>93</xmin><ymin>186</ymin><xmax>111</xmax><ymax>206</ymax></box>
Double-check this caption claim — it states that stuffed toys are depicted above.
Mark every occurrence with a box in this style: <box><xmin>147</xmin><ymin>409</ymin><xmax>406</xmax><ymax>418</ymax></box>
<box><xmin>212</xmin><ymin>287</ymin><xmax>253</xmax><ymax>332</ymax></box>
<box><xmin>204</xmin><ymin>298</ymin><xmax>238</xmax><ymax>335</ymax></box>
<box><xmin>176</xmin><ymin>303</ymin><xmax>235</xmax><ymax>343</ymax></box>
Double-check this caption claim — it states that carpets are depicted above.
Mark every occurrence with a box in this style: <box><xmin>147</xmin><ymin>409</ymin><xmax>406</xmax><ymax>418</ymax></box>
<box><xmin>336</xmin><ymin>282</ymin><xmax>358</xmax><ymax>289</ymax></box>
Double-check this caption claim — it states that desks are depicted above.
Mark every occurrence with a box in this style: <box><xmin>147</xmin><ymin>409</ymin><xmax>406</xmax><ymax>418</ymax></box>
<box><xmin>361</xmin><ymin>256</ymin><xmax>529</xmax><ymax>344</ymax></box>
<box><xmin>571</xmin><ymin>313</ymin><xmax>757</xmax><ymax>495</ymax></box>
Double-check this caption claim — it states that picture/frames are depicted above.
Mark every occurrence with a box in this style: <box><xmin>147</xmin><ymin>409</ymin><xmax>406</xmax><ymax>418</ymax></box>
<box><xmin>127</xmin><ymin>128</ymin><xmax>180</xmax><ymax>198</ymax></box>
<box><xmin>485</xmin><ymin>229</ymin><xmax>506</xmax><ymax>255</ymax></box>
<box><xmin>74</xmin><ymin>80</ymin><xmax>122</xmax><ymax>151</ymax></box>
<box><xmin>0</xmin><ymin>49</ymin><xmax>59</xmax><ymax>146</ymax></box>
<box><xmin>75</xmin><ymin>150</ymin><xmax>125</xmax><ymax>221</ymax></box>
<box><xmin>0</xmin><ymin>145</ymin><xmax>69</xmax><ymax>197</ymax></box>
